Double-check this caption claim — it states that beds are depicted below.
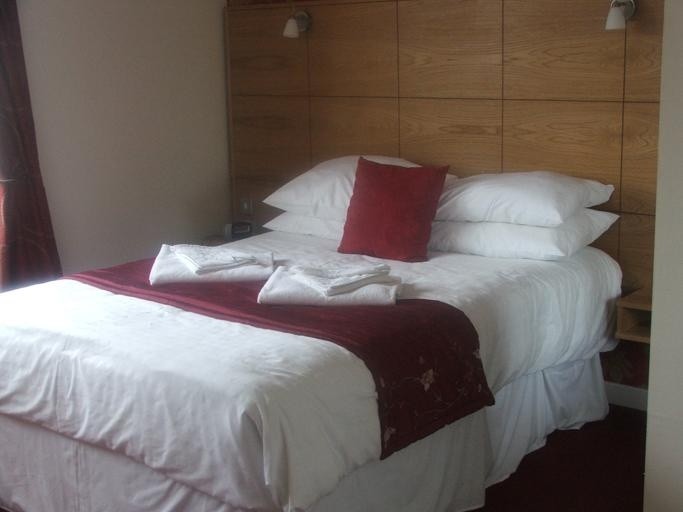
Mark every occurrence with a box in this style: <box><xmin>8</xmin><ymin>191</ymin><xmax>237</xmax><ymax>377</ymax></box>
<box><xmin>0</xmin><ymin>232</ymin><xmax>622</xmax><ymax>512</ymax></box>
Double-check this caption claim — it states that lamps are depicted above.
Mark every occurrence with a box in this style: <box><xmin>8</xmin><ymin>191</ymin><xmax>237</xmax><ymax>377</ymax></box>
<box><xmin>605</xmin><ymin>0</ymin><xmax>636</xmax><ymax>30</ymax></box>
<box><xmin>283</xmin><ymin>11</ymin><xmax>309</xmax><ymax>38</ymax></box>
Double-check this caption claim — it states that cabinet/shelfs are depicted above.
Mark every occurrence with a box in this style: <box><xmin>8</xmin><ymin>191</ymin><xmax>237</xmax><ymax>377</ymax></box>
<box><xmin>615</xmin><ymin>286</ymin><xmax>652</xmax><ymax>344</ymax></box>
<box><xmin>200</xmin><ymin>234</ymin><xmax>233</xmax><ymax>246</ymax></box>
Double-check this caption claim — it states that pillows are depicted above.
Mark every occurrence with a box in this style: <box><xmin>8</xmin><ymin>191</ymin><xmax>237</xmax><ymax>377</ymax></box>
<box><xmin>435</xmin><ymin>170</ymin><xmax>615</xmax><ymax>228</ymax></box>
<box><xmin>263</xmin><ymin>212</ymin><xmax>347</xmax><ymax>241</ymax></box>
<box><xmin>427</xmin><ymin>208</ymin><xmax>620</xmax><ymax>261</ymax></box>
<box><xmin>337</xmin><ymin>156</ymin><xmax>449</xmax><ymax>262</ymax></box>
<box><xmin>262</xmin><ymin>156</ymin><xmax>456</xmax><ymax>213</ymax></box>
